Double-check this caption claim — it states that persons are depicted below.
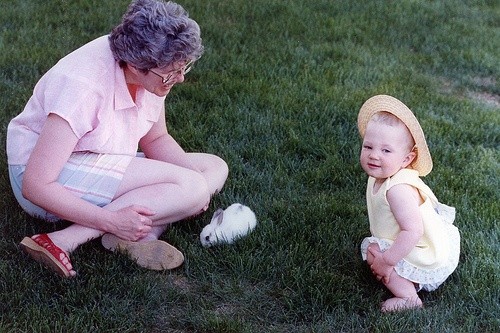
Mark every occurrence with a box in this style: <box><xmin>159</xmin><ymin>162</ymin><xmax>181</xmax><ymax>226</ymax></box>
<box><xmin>7</xmin><ymin>0</ymin><xmax>229</xmax><ymax>280</ymax></box>
<box><xmin>357</xmin><ymin>95</ymin><xmax>460</xmax><ymax>312</ymax></box>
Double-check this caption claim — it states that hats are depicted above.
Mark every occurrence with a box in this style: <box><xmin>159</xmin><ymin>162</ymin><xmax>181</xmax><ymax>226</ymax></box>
<box><xmin>358</xmin><ymin>94</ymin><xmax>433</xmax><ymax>177</ymax></box>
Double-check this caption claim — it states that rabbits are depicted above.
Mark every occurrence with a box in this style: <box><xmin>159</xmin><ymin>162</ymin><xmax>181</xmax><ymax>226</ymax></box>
<box><xmin>200</xmin><ymin>203</ymin><xmax>257</xmax><ymax>249</ymax></box>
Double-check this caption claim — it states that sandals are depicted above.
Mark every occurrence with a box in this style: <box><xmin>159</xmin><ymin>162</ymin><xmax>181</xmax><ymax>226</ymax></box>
<box><xmin>20</xmin><ymin>232</ymin><xmax>73</xmax><ymax>278</ymax></box>
<box><xmin>101</xmin><ymin>232</ymin><xmax>184</xmax><ymax>269</ymax></box>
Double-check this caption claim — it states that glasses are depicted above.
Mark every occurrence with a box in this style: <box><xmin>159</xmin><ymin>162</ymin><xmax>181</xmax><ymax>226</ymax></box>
<box><xmin>147</xmin><ymin>59</ymin><xmax>195</xmax><ymax>83</ymax></box>
